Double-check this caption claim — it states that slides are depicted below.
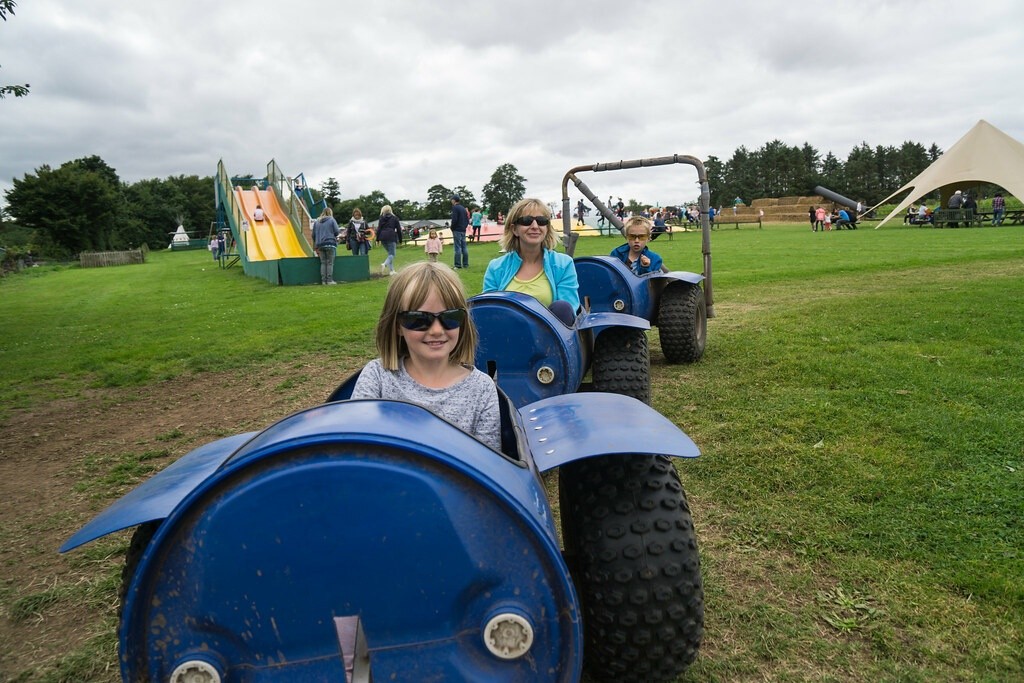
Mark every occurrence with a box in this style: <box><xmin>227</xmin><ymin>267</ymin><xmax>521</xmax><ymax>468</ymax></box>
<box><xmin>223</xmin><ymin>185</ymin><xmax>311</xmax><ymax>263</ymax></box>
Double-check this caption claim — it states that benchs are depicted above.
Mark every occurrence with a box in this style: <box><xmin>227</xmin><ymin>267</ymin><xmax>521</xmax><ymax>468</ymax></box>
<box><xmin>832</xmin><ymin>220</ymin><xmax>862</xmax><ymax>229</ymax></box>
<box><xmin>647</xmin><ymin>223</ymin><xmax>673</xmax><ymax>241</ymax></box>
<box><xmin>904</xmin><ymin>209</ymin><xmax>1024</xmax><ymax>229</ymax></box>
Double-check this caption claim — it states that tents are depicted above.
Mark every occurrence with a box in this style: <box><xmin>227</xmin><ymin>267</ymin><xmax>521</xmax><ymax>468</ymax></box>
<box><xmin>856</xmin><ymin>119</ymin><xmax>1024</xmax><ymax>230</ymax></box>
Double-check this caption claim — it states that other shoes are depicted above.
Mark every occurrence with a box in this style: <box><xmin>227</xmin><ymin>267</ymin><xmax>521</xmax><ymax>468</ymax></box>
<box><xmin>389</xmin><ymin>271</ymin><xmax>395</xmax><ymax>275</ymax></box>
<box><xmin>381</xmin><ymin>263</ymin><xmax>386</xmax><ymax>275</ymax></box>
<box><xmin>454</xmin><ymin>266</ymin><xmax>461</xmax><ymax>270</ymax></box>
<box><xmin>322</xmin><ymin>281</ymin><xmax>337</xmax><ymax>285</ymax></box>
<box><xmin>499</xmin><ymin>249</ymin><xmax>506</xmax><ymax>253</ymax></box>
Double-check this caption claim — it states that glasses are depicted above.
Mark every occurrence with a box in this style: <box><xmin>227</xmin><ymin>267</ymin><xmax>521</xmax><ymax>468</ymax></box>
<box><xmin>626</xmin><ymin>234</ymin><xmax>648</xmax><ymax>241</ymax></box>
<box><xmin>396</xmin><ymin>309</ymin><xmax>470</xmax><ymax>331</ymax></box>
<box><xmin>516</xmin><ymin>216</ymin><xmax>549</xmax><ymax>226</ymax></box>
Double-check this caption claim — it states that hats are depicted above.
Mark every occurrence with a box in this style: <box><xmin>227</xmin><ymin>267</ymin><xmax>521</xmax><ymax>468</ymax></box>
<box><xmin>451</xmin><ymin>194</ymin><xmax>460</xmax><ymax>200</ymax></box>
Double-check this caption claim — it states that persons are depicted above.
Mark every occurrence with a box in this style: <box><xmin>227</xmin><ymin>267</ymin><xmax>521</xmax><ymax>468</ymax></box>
<box><xmin>482</xmin><ymin>198</ymin><xmax>580</xmax><ymax>325</ymax></box>
<box><xmin>412</xmin><ymin>207</ymin><xmax>506</xmax><ymax>244</ymax></box>
<box><xmin>577</xmin><ymin>199</ymin><xmax>586</xmax><ymax>225</ymax></box>
<box><xmin>294</xmin><ymin>178</ymin><xmax>304</xmax><ymax>201</ymax></box>
<box><xmin>425</xmin><ymin>229</ymin><xmax>443</xmax><ymax>261</ymax></box>
<box><xmin>450</xmin><ymin>195</ymin><xmax>469</xmax><ymax>269</ymax></box>
<box><xmin>809</xmin><ymin>206</ymin><xmax>858</xmax><ymax>232</ymax></box>
<box><xmin>210</xmin><ymin>232</ymin><xmax>225</xmax><ymax>261</ymax></box>
<box><xmin>346</xmin><ymin>208</ymin><xmax>370</xmax><ymax>255</ymax></box>
<box><xmin>254</xmin><ymin>205</ymin><xmax>264</xmax><ymax>221</ymax></box>
<box><xmin>610</xmin><ymin>215</ymin><xmax>662</xmax><ymax>276</ymax></box>
<box><xmin>350</xmin><ymin>261</ymin><xmax>502</xmax><ymax>454</ymax></box>
<box><xmin>311</xmin><ymin>208</ymin><xmax>340</xmax><ymax>284</ymax></box>
<box><xmin>903</xmin><ymin>190</ymin><xmax>977</xmax><ymax>228</ymax></box>
<box><xmin>598</xmin><ymin>196</ymin><xmax>764</xmax><ymax>241</ymax></box>
<box><xmin>376</xmin><ymin>205</ymin><xmax>403</xmax><ymax>275</ymax></box>
<box><xmin>991</xmin><ymin>193</ymin><xmax>1005</xmax><ymax>227</ymax></box>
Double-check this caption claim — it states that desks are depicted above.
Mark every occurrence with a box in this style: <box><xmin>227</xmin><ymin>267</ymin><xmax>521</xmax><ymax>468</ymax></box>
<box><xmin>1004</xmin><ymin>210</ymin><xmax>1024</xmax><ymax>225</ymax></box>
<box><xmin>909</xmin><ymin>210</ymin><xmax>934</xmax><ymax>215</ymax></box>
<box><xmin>976</xmin><ymin>212</ymin><xmax>997</xmax><ymax>219</ymax></box>
